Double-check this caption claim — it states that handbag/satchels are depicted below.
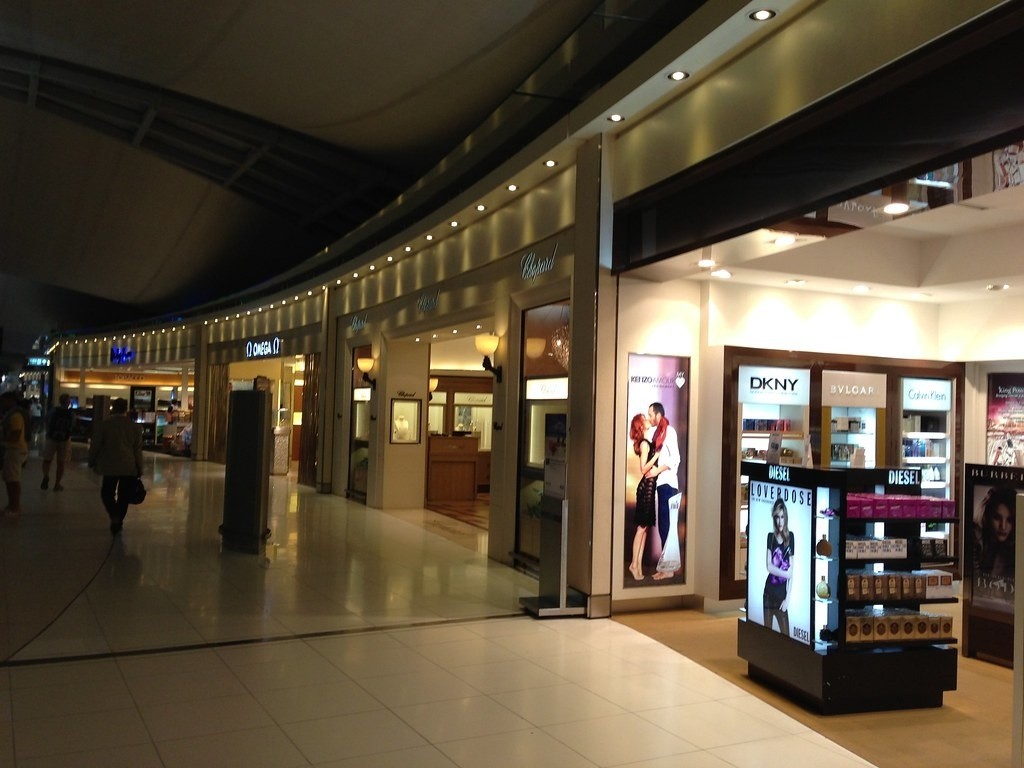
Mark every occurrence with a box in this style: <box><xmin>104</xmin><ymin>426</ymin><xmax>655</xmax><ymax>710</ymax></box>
<box><xmin>118</xmin><ymin>477</ymin><xmax>146</xmax><ymax>504</ymax></box>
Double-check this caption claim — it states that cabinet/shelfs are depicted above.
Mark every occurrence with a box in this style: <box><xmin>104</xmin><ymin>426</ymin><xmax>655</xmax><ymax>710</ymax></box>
<box><xmin>736</xmin><ymin>459</ymin><xmax>960</xmax><ymax>712</ymax></box>
<box><xmin>734</xmin><ymin>429</ymin><xmax>950</xmax><ymax>582</ymax></box>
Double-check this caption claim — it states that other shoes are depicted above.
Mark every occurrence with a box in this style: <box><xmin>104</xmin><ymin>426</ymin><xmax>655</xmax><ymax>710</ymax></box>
<box><xmin>53</xmin><ymin>484</ymin><xmax>64</xmax><ymax>491</ymax></box>
<box><xmin>628</xmin><ymin>563</ymin><xmax>645</xmax><ymax>582</ymax></box>
<box><xmin>41</xmin><ymin>475</ymin><xmax>49</xmax><ymax>490</ymax></box>
<box><xmin>1</xmin><ymin>509</ymin><xmax>22</xmax><ymax>516</ymax></box>
<box><xmin>651</xmin><ymin>571</ymin><xmax>675</xmax><ymax>582</ymax></box>
<box><xmin>111</xmin><ymin>522</ymin><xmax>123</xmax><ymax>536</ymax></box>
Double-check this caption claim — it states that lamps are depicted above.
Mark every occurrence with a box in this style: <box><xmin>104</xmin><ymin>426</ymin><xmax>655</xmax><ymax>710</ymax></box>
<box><xmin>474</xmin><ymin>335</ymin><xmax>502</xmax><ymax>383</ymax></box>
<box><xmin>428</xmin><ymin>378</ymin><xmax>438</xmax><ymax>401</ymax></box>
<box><xmin>357</xmin><ymin>358</ymin><xmax>376</xmax><ymax>390</ymax></box>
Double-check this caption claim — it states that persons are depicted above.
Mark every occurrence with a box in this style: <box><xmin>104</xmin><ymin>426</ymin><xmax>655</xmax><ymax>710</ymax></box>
<box><xmin>974</xmin><ymin>485</ymin><xmax>1017</xmax><ymax>601</ymax></box>
<box><xmin>764</xmin><ymin>498</ymin><xmax>794</xmax><ymax>637</ymax></box>
<box><xmin>88</xmin><ymin>398</ymin><xmax>144</xmax><ymax>532</ymax></box>
<box><xmin>41</xmin><ymin>393</ymin><xmax>74</xmax><ymax>491</ymax></box>
<box><xmin>630</xmin><ymin>402</ymin><xmax>681</xmax><ymax>580</ymax></box>
<box><xmin>0</xmin><ymin>391</ymin><xmax>46</xmax><ymax>516</ymax></box>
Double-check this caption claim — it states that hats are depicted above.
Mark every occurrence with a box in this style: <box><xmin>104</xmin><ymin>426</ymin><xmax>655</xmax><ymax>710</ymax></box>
<box><xmin>113</xmin><ymin>398</ymin><xmax>127</xmax><ymax>405</ymax></box>
<box><xmin>59</xmin><ymin>394</ymin><xmax>69</xmax><ymax>400</ymax></box>
